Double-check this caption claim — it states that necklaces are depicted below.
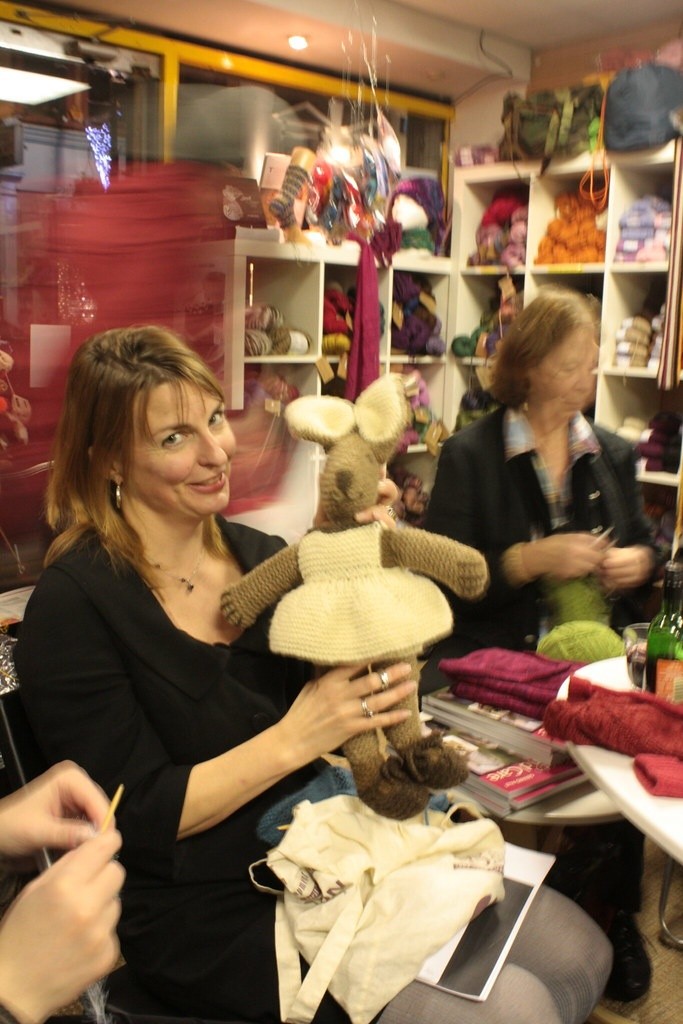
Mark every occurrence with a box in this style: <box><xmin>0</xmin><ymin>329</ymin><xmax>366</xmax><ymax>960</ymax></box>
<box><xmin>140</xmin><ymin>539</ymin><xmax>212</xmax><ymax>591</ymax></box>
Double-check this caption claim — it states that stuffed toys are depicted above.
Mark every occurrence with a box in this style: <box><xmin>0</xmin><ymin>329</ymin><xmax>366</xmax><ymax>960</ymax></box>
<box><xmin>214</xmin><ymin>370</ymin><xmax>489</xmax><ymax>822</ymax></box>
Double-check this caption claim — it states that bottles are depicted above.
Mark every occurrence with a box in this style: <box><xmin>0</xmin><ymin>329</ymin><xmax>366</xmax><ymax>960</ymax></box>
<box><xmin>646</xmin><ymin>560</ymin><xmax>683</xmax><ymax>705</ymax></box>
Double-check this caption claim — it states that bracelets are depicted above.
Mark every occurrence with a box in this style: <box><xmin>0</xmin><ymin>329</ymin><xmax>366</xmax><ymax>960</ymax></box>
<box><xmin>505</xmin><ymin>542</ymin><xmax>535</xmax><ymax>585</ymax></box>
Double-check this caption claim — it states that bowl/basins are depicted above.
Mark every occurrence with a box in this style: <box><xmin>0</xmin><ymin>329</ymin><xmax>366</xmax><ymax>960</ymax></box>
<box><xmin>623</xmin><ymin>622</ymin><xmax>651</xmax><ymax>688</ymax></box>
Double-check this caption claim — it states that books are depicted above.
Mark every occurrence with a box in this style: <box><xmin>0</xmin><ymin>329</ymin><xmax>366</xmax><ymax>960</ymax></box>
<box><xmin>414</xmin><ymin>684</ymin><xmax>590</xmax><ymax>819</ymax></box>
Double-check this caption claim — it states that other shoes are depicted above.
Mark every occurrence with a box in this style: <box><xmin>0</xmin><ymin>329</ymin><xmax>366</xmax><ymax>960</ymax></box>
<box><xmin>597</xmin><ymin>910</ymin><xmax>653</xmax><ymax>1016</ymax></box>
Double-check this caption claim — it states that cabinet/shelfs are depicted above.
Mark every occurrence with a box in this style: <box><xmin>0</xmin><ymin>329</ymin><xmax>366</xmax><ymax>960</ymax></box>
<box><xmin>221</xmin><ymin>134</ymin><xmax>683</xmax><ymax>622</ymax></box>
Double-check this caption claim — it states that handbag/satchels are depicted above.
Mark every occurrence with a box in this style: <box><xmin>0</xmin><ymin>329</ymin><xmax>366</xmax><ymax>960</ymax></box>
<box><xmin>250</xmin><ymin>795</ymin><xmax>505</xmax><ymax>1024</ymax></box>
<box><xmin>497</xmin><ymin>83</ymin><xmax>604</xmax><ymax>185</ymax></box>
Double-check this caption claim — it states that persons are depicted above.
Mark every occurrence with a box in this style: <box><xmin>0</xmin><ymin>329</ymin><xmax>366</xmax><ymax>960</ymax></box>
<box><xmin>423</xmin><ymin>286</ymin><xmax>668</xmax><ymax>1007</ymax></box>
<box><xmin>385</xmin><ymin>177</ymin><xmax>444</xmax><ymax>257</ymax></box>
<box><xmin>9</xmin><ymin>327</ymin><xmax>618</xmax><ymax>1024</ymax></box>
<box><xmin>0</xmin><ymin>759</ymin><xmax>126</xmax><ymax>1023</ymax></box>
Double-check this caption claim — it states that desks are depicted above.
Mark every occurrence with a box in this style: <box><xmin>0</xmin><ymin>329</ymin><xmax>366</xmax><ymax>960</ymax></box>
<box><xmin>419</xmin><ymin>656</ymin><xmax>683</xmax><ymax>949</ymax></box>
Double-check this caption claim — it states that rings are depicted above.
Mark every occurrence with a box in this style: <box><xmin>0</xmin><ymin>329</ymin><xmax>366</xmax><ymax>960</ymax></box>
<box><xmin>361</xmin><ymin>699</ymin><xmax>374</xmax><ymax>719</ymax></box>
<box><xmin>376</xmin><ymin>667</ymin><xmax>389</xmax><ymax>691</ymax></box>
